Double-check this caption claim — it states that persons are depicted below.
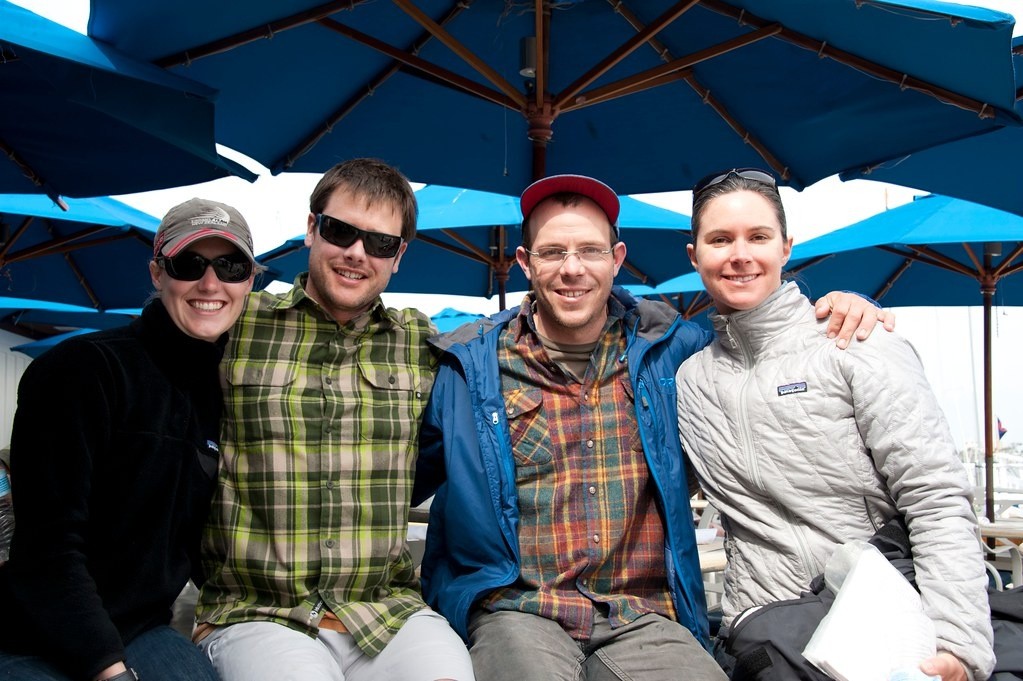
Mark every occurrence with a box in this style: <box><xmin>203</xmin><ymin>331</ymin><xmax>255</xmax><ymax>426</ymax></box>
<box><xmin>0</xmin><ymin>2</ymin><xmax>1023</xmax><ymax>560</ymax></box>
<box><xmin>676</xmin><ymin>168</ymin><xmax>998</xmax><ymax>681</ymax></box>
<box><xmin>187</xmin><ymin>157</ymin><xmax>476</xmax><ymax>681</ymax></box>
<box><xmin>0</xmin><ymin>199</ymin><xmax>269</xmax><ymax>681</ymax></box>
<box><xmin>402</xmin><ymin>172</ymin><xmax>895</xmax><ymax>681</ymax></box>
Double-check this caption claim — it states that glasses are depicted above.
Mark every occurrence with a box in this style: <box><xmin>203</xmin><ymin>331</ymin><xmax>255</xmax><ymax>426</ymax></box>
<box><xmin>692</xmin><ymin>168</ymin><xmax>780</xmax><ymax>199</ymax></box>
<box><xmin>524</xmin><ymin>246</ymin><xmax>615</xmax><ymax>267</ymax></box>
<box><xmin>315</xmin><ymin>212</ymin><xmax>405</xmax><ymax>259</ymax></box>
<box><xmin>156</xmin><ymin>251</ymin><xmax>253</xmax><ymax>283</ymax></box>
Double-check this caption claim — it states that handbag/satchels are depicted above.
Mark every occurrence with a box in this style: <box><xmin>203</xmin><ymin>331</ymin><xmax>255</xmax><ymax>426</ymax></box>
<box><xmin>721</xmin><ymin>519</ymin><xmax>1023</xmax><ymax>681</ymax></box>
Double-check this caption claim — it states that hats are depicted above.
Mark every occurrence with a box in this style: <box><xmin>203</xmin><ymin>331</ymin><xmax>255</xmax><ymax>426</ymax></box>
<box><xmin>153</xmin><ymin>197</ymin><xmax>254</xmax><ymax>271</ymax></box>
<box><xmin>521</xmin><ymin>174</ymin><xmax>620</xmax><ymax>239</ymax></box>
